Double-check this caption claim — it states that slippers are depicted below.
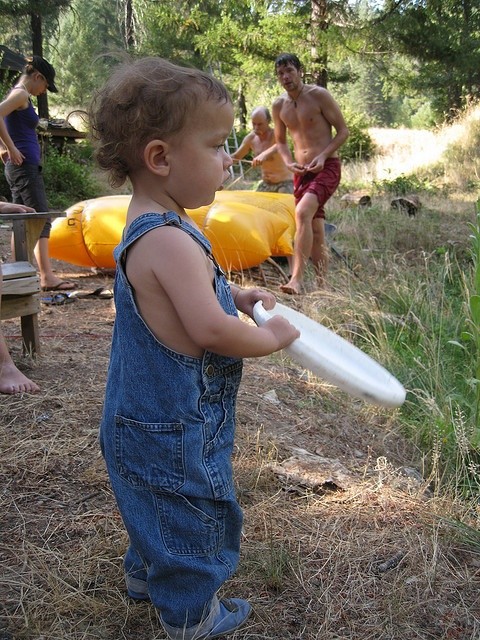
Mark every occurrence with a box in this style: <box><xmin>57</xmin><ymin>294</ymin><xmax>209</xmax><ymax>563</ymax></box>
<box><xmin>75</xmin><ymin>287</ymin><xmax>114</xmax><ymax>299</ymax></box>
<box><xmin>41</xmin><ymin>281</ymin><xmax>78</xmax><ymax>291</ymax></box>
<box><xmin>42</xmin><ymin>292</ymin><xmax>77</xmax><ymax>306</ymax></box>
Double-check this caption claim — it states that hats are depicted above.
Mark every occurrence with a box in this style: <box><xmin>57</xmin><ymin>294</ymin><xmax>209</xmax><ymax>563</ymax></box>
<box><xmin>27</xmin><ymin>55</ymin><xmax>58</xmax><ymax>93</ymax></box>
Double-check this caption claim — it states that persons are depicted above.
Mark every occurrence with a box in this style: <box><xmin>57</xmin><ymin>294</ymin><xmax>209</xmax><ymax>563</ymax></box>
<box><xmin>275</xmin><ymin>55</ymin><xmax>347</xmax><ymax>295</ymax></box>
<box><xmin>89</xmin><ymin>59</ymin><xmax>299</xmax><ymax>638</ymax></box>
<box><xmin>0</xmin><ymin>58</ymin><xmax>77</xmax><ymax>290</ymax></box>
<box><xmin>0</xmin><ymin>201</ymin><xmax>40</xmax><ymax>394</ymax></box>
<box><xmin>230</xmin><ymin>107</ymin><xmax>295</xmax><ymax>194</ymax></box>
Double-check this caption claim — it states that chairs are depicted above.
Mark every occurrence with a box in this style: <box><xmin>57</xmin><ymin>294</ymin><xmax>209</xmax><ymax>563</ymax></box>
<box><xmin>0</xmin><ymin>211</ymin><xmax>67</xmax><ymax>358</ymax></box>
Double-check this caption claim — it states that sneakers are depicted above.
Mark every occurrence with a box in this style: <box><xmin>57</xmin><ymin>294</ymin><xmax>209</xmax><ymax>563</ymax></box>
<box><xmin>167</xmin><ymin>598</ymin><xmax>252</xmax><ymax>640</ymax></box>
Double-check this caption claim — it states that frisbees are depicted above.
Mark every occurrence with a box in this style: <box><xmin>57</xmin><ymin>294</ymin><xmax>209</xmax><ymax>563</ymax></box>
<box><xmin>253</xmin><ymin>299</ymin><xmax>406</xmax><ymax>408</ymax></box>
